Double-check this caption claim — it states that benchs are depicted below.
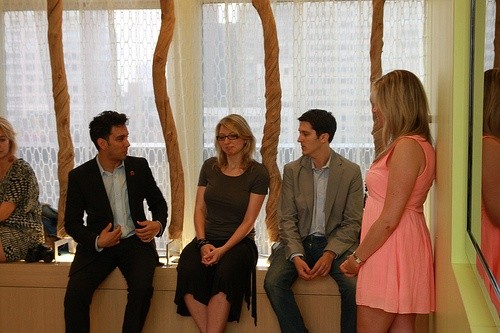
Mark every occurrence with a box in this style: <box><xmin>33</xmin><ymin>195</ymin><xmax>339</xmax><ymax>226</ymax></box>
<box><xmin>1</xmin><ymin>254</ymin><xmax>429</xmax><ymax>333</ymax></box>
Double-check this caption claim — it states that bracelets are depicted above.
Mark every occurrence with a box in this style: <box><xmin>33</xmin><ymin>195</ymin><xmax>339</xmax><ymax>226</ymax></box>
<box><xmin>198</xmin><ymin>239</ymin><xmax>208</xmax><ymax>247</ymax></box>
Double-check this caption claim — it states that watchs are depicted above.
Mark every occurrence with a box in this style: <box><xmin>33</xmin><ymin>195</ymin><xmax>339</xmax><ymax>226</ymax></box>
<box><xmin>353</xmin><ymin>251</ymin><xmax>366</xmax><ymax>265</ymax></box>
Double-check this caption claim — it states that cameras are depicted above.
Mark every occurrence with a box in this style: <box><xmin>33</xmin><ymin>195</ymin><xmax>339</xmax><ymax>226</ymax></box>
<box><xmin>24</xmin><ymin>244</ymin><xmax>55</xmax><ymax>263</ymax></box>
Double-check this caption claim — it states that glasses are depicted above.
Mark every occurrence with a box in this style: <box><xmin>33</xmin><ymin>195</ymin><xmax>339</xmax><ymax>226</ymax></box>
<box><xmin>216</xmin><ymin>134</ymin><xmax>239</xmax><ymax>141</ymax></box>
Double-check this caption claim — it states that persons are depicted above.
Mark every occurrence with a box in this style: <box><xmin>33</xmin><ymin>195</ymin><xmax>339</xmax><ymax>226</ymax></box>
<box><xmin>263</xmin><ymin>110</ymin><xmax>364</xmax><ymax>333</ymax></box>
<box><xmin>1</xmin><ymin>117</ymin><xmax>44</xmax><ymax>264</ymax></box>
<box><xmin>480</xmin><ymin>69</ymin><xmax>500</xmax><ymax>287</ymax></box>
<box><xmin>173</xmin><ymin>115</ymin><xmax>270</xmax><ymax>332</ymax></box>
<box><xmin>63</xmin><ymin>111</ymin><xmax>168</xmax><ymax>333</ymax></box>
<box><xmin>339</xmin><ymin>69</ymin><xmax>436</xmax><ymax>333</ymax></box>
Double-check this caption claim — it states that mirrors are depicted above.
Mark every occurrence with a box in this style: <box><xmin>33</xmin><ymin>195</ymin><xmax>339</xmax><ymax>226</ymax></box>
<box><xmin>468</xmin><ymin>1</ymin><xmax>500</xmax><ymax>300</ymax></box>
